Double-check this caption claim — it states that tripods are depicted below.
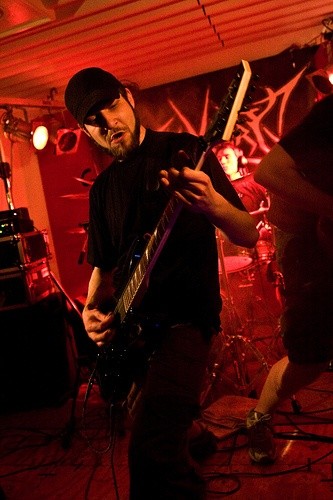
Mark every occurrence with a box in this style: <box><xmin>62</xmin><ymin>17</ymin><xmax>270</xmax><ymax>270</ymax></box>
<box><xmin>195</xmin><ymin>224</ymin><xmax>272</xmax><ymax>411</ymax></box>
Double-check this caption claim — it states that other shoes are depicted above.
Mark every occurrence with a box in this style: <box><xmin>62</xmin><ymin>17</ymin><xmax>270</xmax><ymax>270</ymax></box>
<box><xmin>245</xmin><ymin>408</ymin><xmax>278</xmax><ymax>463</ymax></box>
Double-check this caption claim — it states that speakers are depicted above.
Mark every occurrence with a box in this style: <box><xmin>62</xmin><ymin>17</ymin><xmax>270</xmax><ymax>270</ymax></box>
<box><xmin>0</xmin><ymin>291</ymin><xmax>71</xmax><ymax>411</ymax></box>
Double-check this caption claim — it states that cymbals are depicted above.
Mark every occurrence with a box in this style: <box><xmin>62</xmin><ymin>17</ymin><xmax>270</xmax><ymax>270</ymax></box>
<box><xmin>65</xmin><ymin>227</ymin><xmax>86</xmax><ymax>233</ymax></box>
<box><xmin>61</xmin><ymin>192</ymin><xmax>89</xmax><ymax>200</ymax></box>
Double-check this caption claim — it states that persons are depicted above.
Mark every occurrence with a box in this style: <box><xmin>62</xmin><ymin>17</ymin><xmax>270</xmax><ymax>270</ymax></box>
<box><xmin>246</xmin><ymin>85</ymin><xmax>333</xmax><ymax>464</ymax></box>
<box><xmin>63</xmin><ymin>62</ymin><xmax>259</xmax><ymax>500</ymax></box>
<box><xmin>213</xmin><ymin>141</ymin><xmax>269</xmax><ymax>251</ymax></box>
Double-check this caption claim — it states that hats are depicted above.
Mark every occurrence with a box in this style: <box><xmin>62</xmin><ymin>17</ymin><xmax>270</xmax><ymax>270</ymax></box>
<box><xmin>65</xmin><ymin>68</ymin><xmax>120</xmax><ymax>128</ymax></box>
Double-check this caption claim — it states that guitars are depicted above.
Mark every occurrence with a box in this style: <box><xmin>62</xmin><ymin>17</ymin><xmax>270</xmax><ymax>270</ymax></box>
<box><xmin>84</xmin><ymin>59</ymin><xmax>252</xmax><ymax>385</ymax></box>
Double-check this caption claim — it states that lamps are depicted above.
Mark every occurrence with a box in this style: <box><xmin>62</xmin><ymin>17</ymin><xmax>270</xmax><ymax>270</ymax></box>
<box><xmin>305</xmin><ymin>65</ymin><xmax>333</xmax><ymax>103</ymax></box>
<box><xmin>32</xmin><ymin>117</ymin><xmax>81</xmax><ymax>155</ymax></box>
<box><xmin>2</xmin><ymin>116</ymin><xmax>50</xmax><ymax>150</ymax></box>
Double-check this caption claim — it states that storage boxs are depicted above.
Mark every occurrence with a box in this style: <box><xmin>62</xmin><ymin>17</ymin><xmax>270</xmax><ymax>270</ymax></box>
<box><xmin>0</xmin><ymin>229</ymin><xmax>55</xmax><ymax>310</ymax></box>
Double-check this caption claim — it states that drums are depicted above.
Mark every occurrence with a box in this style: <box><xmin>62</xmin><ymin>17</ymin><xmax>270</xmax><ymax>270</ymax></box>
<box><xmin>218</xmin><ymin>256</ymin><xmax>257</xmax><ymax>300</ymax></box>
<box><xmin>240</xmin><ymin>240</ymin><xmax>282</xmax><ymax>292</ymax></box>
